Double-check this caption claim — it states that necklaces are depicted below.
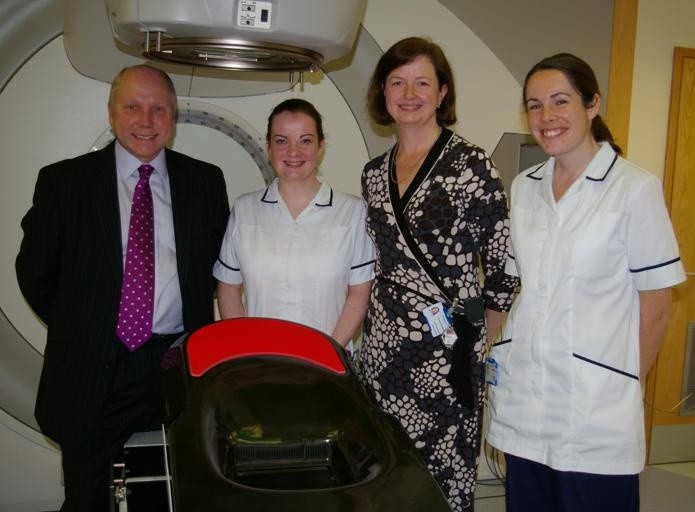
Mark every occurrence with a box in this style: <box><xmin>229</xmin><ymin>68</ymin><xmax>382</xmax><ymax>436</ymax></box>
<box><xmin>390</xmin><ymin>142</ymin><xmax>432</xmax><ymax>186</ymax></box>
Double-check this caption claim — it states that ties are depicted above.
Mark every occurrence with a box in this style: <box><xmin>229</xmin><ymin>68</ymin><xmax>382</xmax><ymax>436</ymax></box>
<box><xmin>116</xmin><ymin>164</ymin><xmax>155</xmax><ymax>353</ymax></box>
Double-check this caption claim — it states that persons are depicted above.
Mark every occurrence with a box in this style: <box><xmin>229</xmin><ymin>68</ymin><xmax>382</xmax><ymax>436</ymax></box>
<box><xmin>355</xmin><ymin>38</ymin><xmax>521</xmax><ymax>512</ymax></box>
<box><xmin>16</xmin><ymin>66</ymin><xmax>231</xmax><ymax>511</ymax></box>
<box><xmin>487</xmin><ymin>53</ymin><xmax>687</xmax><ymax>512</ymax></box>
<box><xmin>213</xmin><ymin>99</ymin><xmax>375</xmax><ymax>348</ymax></box>
